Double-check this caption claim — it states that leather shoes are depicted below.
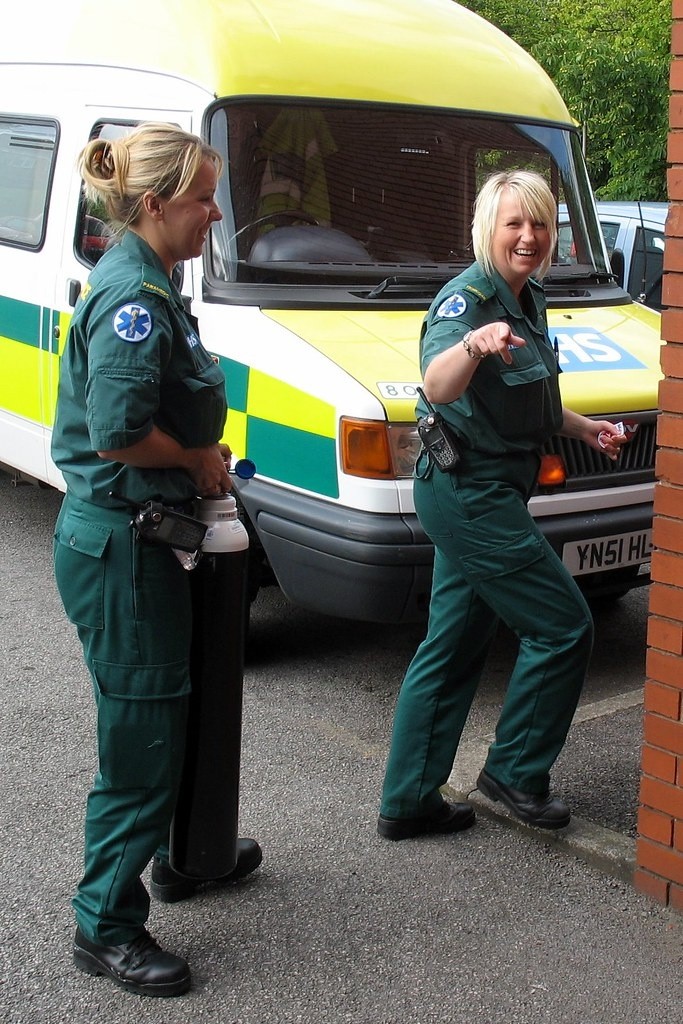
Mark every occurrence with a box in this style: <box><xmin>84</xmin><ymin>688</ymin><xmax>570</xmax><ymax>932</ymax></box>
<box><xmin>149</xmin><ymin>837</ymin><xmax>263</xmax><ymax>904</ymax></box>
<box><xmin>73</xmin><ymin>924</ymin><xmax>191</xmax><ymax>997</ymax></box>
<box><xmin>376</xmin><ymin>798</ymin><xmax>475</xmax><ymax>841</ymax></box>
<box><xmin>476</xmin><ymin>767</ymin><xmax>571</xmax><ymax>830</ymax></box>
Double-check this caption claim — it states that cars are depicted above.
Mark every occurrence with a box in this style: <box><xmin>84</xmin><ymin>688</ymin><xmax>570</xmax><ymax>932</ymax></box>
<box><xmin>556</xmin><ymin>200</ymin><xmax>670</xmax><ymax>309</ymax></box>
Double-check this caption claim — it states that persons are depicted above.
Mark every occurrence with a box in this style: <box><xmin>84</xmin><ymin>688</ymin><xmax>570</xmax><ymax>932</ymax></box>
<box><xmin>51</xmin><ymin>122</ymin><xmax>263</xmax><ymax>997</ymax></box>
<box><xmin>375</xmin><ymin>171</ymin><xmax>627</xmax><ymax>841</ymax></box>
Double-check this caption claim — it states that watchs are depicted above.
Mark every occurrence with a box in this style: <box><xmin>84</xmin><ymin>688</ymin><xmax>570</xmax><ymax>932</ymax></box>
<box><xmin>463</xmin><ymin>330</ymin><xmax>487</xmax><ymax>360</ymax></box>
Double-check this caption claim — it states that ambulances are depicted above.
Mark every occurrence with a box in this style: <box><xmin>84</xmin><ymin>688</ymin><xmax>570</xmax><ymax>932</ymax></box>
<box><xmin>0</xmin><ymin>0</ymin><xmax>668</xmax><ymax>624</ymax></box>
